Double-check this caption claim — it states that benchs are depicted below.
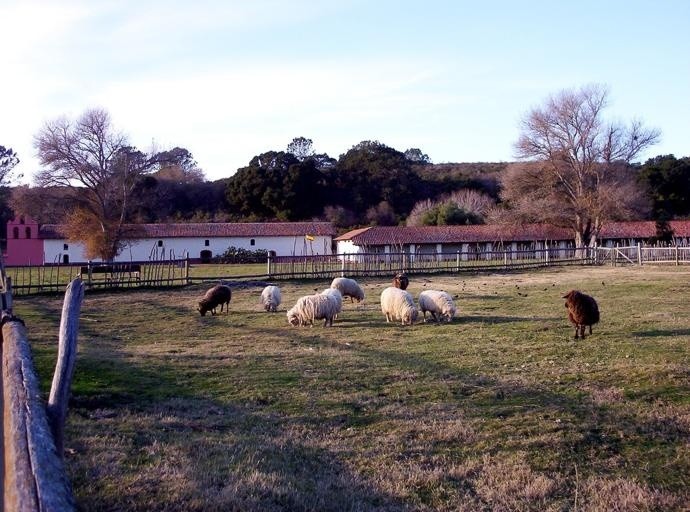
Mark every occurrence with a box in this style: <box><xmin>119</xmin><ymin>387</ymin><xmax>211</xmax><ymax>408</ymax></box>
<box><xmin>80</xmin><ymin>263</ymin><xmax>142</xmax><ymax>287</ymax></box>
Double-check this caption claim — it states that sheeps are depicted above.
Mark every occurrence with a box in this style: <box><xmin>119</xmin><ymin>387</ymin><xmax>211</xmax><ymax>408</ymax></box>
<box><xmin>196</xmin><ymin>283</ymin><xmax>233</xmax><ymax>317</ymax></box>
<box><xmin>417</xmin><ymin>289</ymin><xmax>456</xmax><ymax>325</ymax></box>
<box><xmin>393</xmin><ymin>272</ymin><xmax>409</xmax><ymax>290</ymax></box>
<box><xmin>286</xmin><ymin>292</ymin><xmax>337</xmax><ymax>327</ymax></box>
<box><xmin>321</xmin><ymin>287</ymin><xmax>343</xmax><ymax>321</ymax></box>
<box><xmin>330</xmin><ymin>277</ymin><xmax>365</xmax><ymax>304</ymax></box>
<box><xmin>260</xmin><ymin>286</ymin><xmax>281</xmax><ymax>311</ymax></box>
<box><xmin>379</xmin><ymin>287</ymin><xmax>419</xmax><ymax>326</ymax></box>
<box><xmin>562</xmin><ymin>290</ymin><xmax>601</xmax><ymax>342</ymax></box>
